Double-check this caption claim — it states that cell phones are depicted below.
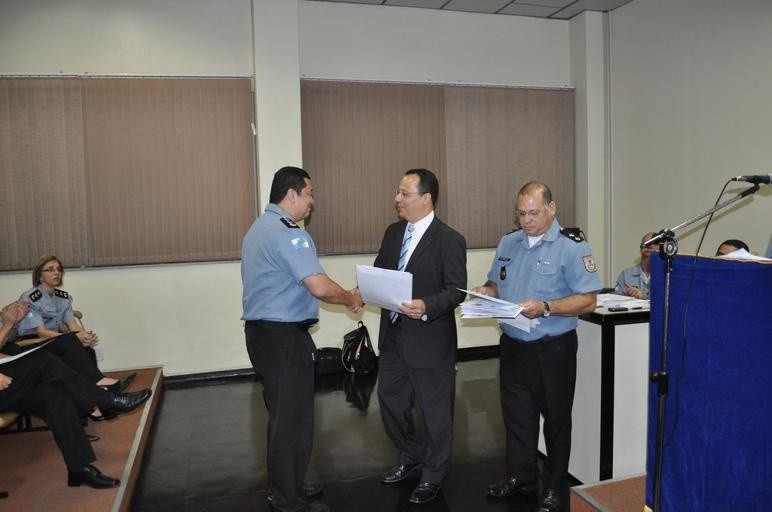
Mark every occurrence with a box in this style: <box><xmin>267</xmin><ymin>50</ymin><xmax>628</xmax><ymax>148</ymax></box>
<box><xmin>608</xmin><ymin>307</ymin><xmax>628</xmax><ymax>312</ymax></box>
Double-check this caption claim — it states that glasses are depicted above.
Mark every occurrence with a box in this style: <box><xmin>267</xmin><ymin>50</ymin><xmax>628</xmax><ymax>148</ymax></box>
<box><xmin>40</xmin><ymin>266</ymin><xmax>64</xmax><ymax>275</ymax></box>
<box><xmin>395</xmin><ymin>189</ymin><xmax>428</xmax><ymax>199</ymax></box>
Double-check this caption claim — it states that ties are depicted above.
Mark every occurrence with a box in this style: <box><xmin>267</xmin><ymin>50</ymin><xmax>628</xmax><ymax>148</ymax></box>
<box><xmin>389</xmin><ymin>224</ymin><xmax>414</xmax><ymax>325</ymax></box>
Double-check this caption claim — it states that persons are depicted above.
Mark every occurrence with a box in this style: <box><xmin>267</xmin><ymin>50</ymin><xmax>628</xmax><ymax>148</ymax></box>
<box><xmin>0</xmin><ymin>301</ymin><xmax>137</xmax><ymax>421</ymax></box>
<box><xmin>14</xmin><ymin>254</ymin><xmax>99</xmax><ymax>368</ymax></box>
<box><xmin>716</xmin><ymin>238</ymin><xmax>750</xmax><ymax>257</ymax></box>
<box><xmin>346</xmin><ymin>169</ymin><xmax>467</xmax><ymax>503</ymax></box>
<box><xmin>614</xmin><ymin>232</ymin><xmax>661</xmax><ymax>301</ymax></box>
<box><xmin>237</xmin><ymin>166</ymin><xmax>366</xmax><ymax>512</ymax></box>
<box><xmin>469</xmin><ymin>179</ymin><xmax>604</xmax><ymax>511</ymax></box>
<box><xmin>0</xmin><ymin>345</ymin><xmax>153</xmax><ymax>489</ymax></box>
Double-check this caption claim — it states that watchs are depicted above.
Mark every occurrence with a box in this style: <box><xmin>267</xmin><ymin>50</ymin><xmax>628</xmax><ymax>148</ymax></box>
<box><xmin>420</xmin><ymin>312</ymin><xmax>428</xmax><ymax>323</ymax></box>
<box><xmin>542</xmin><ymin>300</ymin><xmax>552</xmax><ymax>318</ymax></box>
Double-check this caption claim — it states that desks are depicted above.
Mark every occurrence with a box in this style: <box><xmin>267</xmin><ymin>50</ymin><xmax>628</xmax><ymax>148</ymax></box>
<box><xmin>537</xmin><ymin>306</ymin><xmax>652</xmax><ymax>485</ymax></box>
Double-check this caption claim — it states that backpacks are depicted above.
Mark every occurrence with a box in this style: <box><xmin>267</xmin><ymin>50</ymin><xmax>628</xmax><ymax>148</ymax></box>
<box><xmin>341</xmin><ymin>321</ymin><xmax>378</xmax><ymax>375</ymax></box>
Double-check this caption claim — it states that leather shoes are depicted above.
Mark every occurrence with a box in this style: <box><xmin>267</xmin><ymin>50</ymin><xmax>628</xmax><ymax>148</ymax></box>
<box><xmin>88</xmin><ymin>372</ymin><xmax>151</xmax><ymax>422</ymax></box>
<box><xmin>483</xmin><ymin>475</ymin><xmax>538</xmax><ymax>498</ymax></box>
<box><xmin>67</xmin><ymin>465</ymin><xmax>118</xmax><ymax>489</ymax></box>
<box><xmin>267</xmin><ymin>482</ymin><xmax>325</xmax><ymax>501</ymax></box>
<box><xmin>539</xmin><ymin>488</ymin><xmax>570</xmax><ymax>512</ymax></box>
<box><xmin>381</xmin><ymin>463</ymin><xmax>426</xmax><ymax>483</ymax></box>
<box><xmin>409</xmin><ymin>479</ymin><xmax>443</xmax><ymax>504</ymax></box>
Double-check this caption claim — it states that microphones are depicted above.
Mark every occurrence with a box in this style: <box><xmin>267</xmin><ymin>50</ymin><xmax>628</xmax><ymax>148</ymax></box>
<box><xmin>733</xmin><ymin>174</ymin><xmax>772</xmax><ymax>185</ymax></box>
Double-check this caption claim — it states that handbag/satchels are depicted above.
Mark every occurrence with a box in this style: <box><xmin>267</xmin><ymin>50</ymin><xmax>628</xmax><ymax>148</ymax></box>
<box><xmin>317</xmin><ymin>347</ymin><xmax>344</xmax><ymax>376</ymax></box>
